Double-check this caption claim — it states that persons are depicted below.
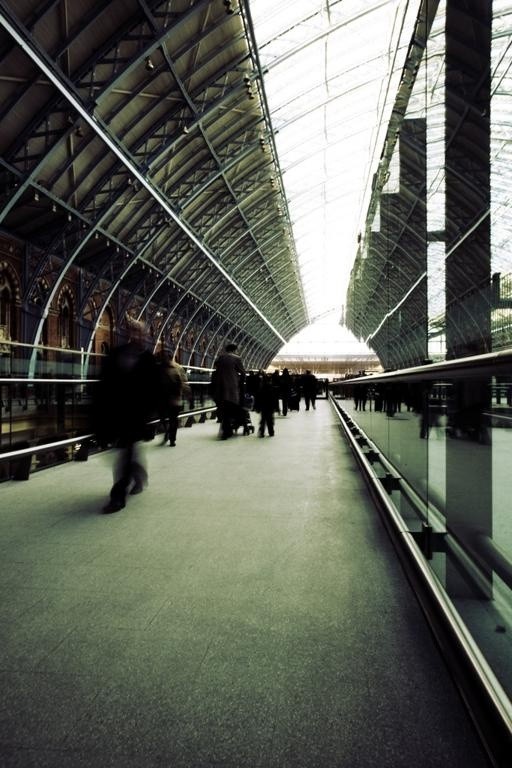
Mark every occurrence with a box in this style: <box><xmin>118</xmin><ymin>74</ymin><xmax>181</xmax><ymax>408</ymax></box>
<box><xmin>332</xmin><ymin>368</ymin><xmax>495</xmax><ymax>446</ymax></box>
<box><xmin>333</xmin><ymin>366</ymin><xmax>406</xmax><ymax>381</ymax></box>
<box><xmin>96</xmin><ymin>317</ymin><xmax>159</xmax><ymax>513</ymax></box>
<box><xmin>209</xmin><ymin>343</ymin><xmax>332</xmax><ymax>447</ymax></box>
<box><xmin>160</xmin><ymin>346</ymin><xmax>189</xmax><ymax>446</ymax></box>
